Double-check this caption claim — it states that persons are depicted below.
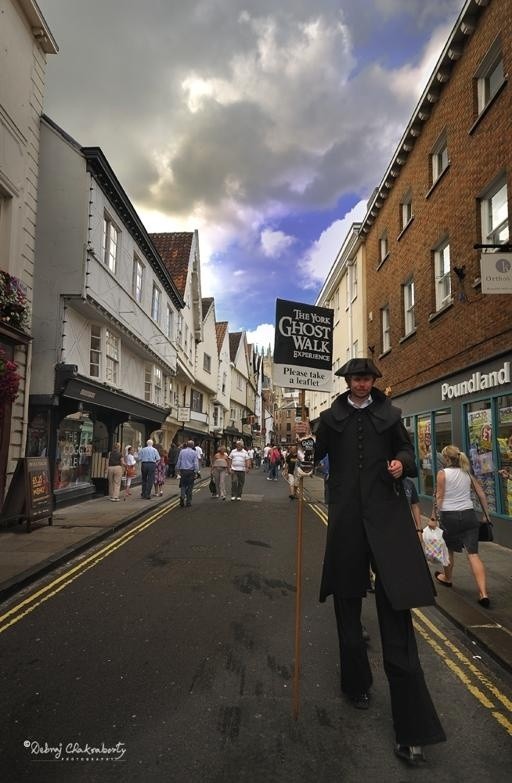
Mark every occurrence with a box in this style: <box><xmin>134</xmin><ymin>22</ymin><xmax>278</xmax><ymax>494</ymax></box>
<box><xmin>429</xmin><ymin>443</ymin><xmax>494</xmax><ymax>606</ymax></box>
<box><xmin>39</xmin><ymin>436</ymin><xmax>329</xmax><ymax>507</ymax></box>
<box><xmin>295</xmin><ymin>358</ymin><xmax>448</xmax><ymax>767</ymax></box>
<box><xmin>400</xmin><ymin>479</ymin><xmax>423</xmax><ymax>542</ymax></box>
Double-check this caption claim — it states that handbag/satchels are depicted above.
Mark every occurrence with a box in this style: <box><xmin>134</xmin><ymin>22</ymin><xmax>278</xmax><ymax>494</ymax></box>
<box><xmin>421</xmin><ymin>526</ymin><xmax>451</xmax><ymax>567</ymax></box>
<box><xmin>209</xmin><ymin>476</ymin><xmax>216</xmax><ymax>495</ymax></box>
<box><xmin>479</xmin><ymin>520</ymin><xmax>494</xmax><ymax>543</ymax></box>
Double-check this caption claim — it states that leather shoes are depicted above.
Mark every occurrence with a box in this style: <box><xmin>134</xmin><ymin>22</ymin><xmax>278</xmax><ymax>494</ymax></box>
<box><xmin>434</xmin><ymin>570</ymin><xmax>453</xmax><ymax>588</ymax></box>
<box><xmin>347</xmin><ymin>691</ymin><xmax>372</xmax><ymax>711</ymax></box>
<box><xmin>395</xmin><ymin>741</ymin><xmax>426</xmax><ymax>766</ymax></box>
<box><xmin>477</xmin><ymin>596</ymin><xmax>490</xmax><ymax>608</ymax></box>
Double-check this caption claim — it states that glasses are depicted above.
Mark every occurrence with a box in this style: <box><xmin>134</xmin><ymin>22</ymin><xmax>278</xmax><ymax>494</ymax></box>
<box><xmin>236</xmin><ymin>444</ymin><xmax>242</xmax><ymax>447</ymax></box>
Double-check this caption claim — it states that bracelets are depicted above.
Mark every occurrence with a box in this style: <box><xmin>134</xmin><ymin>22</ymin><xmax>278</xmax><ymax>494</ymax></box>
<box><xmin>416</xmin><ymin>528</ymin><xmax>423</xmax><ymax>532</ymax></box>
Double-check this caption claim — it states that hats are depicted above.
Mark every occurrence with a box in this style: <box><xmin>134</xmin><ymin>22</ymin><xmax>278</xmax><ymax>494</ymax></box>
<box><xmin>335</xmin><ymin>356</ymin><xmax>382</xmax><ymax>379</ymax></box>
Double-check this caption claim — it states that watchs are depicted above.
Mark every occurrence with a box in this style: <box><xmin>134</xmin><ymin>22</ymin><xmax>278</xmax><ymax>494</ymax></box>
<box><xmin>430</xmin><ymin>517</ymin><xmax>436</xmax><ymax>520</ymax></box>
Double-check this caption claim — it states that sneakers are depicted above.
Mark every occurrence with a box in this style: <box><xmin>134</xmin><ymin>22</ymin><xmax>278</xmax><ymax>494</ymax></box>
<box><xmin>237</xmin><ymin>496</ymin><xmax>241</xmax><ymax>501</ymax></box>
<box><xmin>230</xmin><ymin>497</ymin><xmax>236</xmax><ymax>501</ymax></box>
